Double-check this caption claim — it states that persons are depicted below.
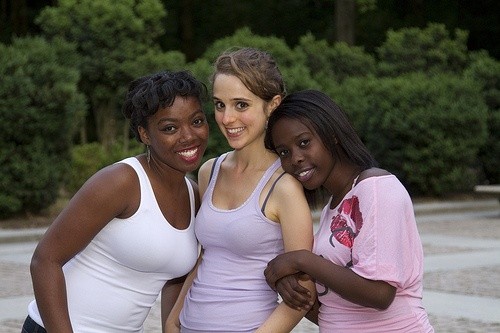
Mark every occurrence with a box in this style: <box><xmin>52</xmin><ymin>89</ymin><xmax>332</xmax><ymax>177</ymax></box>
<box><xmin>264</xmin><ymin>89</ymin><xmax>434</xmax><ymax>333</ymax></box>
<box><xmin>21</xmin><ymin>69</ymin><xmax>210</xmax><ymax>333</ymax></box>
<box><xmin>165</xmin><ymin>47</ymin><xmax>316</xmax><ymax>332</ymax></box>
